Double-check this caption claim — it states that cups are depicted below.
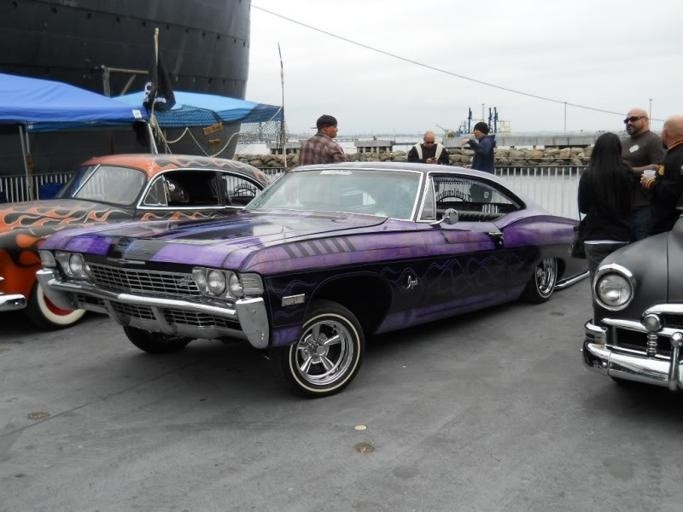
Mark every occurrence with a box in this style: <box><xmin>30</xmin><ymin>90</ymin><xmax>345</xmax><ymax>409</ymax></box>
<box><xmin>644</xmin><ymin>170</ymin><xmax>656</xmax><ymax>177</ymax></box>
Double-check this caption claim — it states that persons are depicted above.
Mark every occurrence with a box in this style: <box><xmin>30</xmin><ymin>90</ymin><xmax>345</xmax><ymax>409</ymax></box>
<box><xmin>637</xmin><ymin>115</ymin><xmax>683</xmax><ymax>237</ymax></box>
<box><xmin>297</xmin><ymin>115</ymin><xmax>347</xmax><ymax>165</ymax></box>
<box><xmin>458</xmin><ymin>122</ymin><xmax>495</xmax><ymax>203</ymax></box>
<box><xmin>574</xmin><ymin>132</ymin><xmax>636</xmax><ymax>324</ymax></box>
<box><xmin>616</xmin><ymin>106</ymin><xmax>663</xmax><ymax>245</ymax></box>
<box><xmin>404</xmin><ymin>130</ymin><xmax>449</xmax><ymax>168</ymax></box>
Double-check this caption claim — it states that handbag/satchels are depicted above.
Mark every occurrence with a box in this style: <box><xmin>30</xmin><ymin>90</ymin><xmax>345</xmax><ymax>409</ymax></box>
<box><xmin>571</xmin><ymin>221</ymin><xmax>587</xmax><ymax>259</ymax></box>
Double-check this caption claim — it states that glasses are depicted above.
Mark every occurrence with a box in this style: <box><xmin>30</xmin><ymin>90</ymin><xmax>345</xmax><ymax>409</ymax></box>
<box><xmin>425</xmin><ymin>141</ymin><xmax>434</xmax><ymax>144</ymax></box>
<box><xmin>624</xmin><ymin>116</ymin><xmax>647</xmax><ymax>123</ymax></box>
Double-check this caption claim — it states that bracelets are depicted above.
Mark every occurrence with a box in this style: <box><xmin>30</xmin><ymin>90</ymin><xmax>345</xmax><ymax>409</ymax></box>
<box><xmin>644</xmin><ymin>179</ymin><xmax>652</xmax><ymax>188</ymax></box>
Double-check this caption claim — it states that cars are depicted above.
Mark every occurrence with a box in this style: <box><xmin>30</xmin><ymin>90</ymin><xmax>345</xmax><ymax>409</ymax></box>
<box><xmin>582</xmin><ymin>212</ymin><xmax>683</xmax><ymax>392</ymax></box>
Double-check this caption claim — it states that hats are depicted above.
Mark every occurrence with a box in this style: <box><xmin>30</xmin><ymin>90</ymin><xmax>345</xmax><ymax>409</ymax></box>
<box><xmin>474</xmin><ymin>122</ymin><xmax>489</xmax><ymax>134</ymax></box>
<box><xmin>316</xmin><ymin>115</ymin><xmax>337</xmax><ymax>128</ymax></box>
